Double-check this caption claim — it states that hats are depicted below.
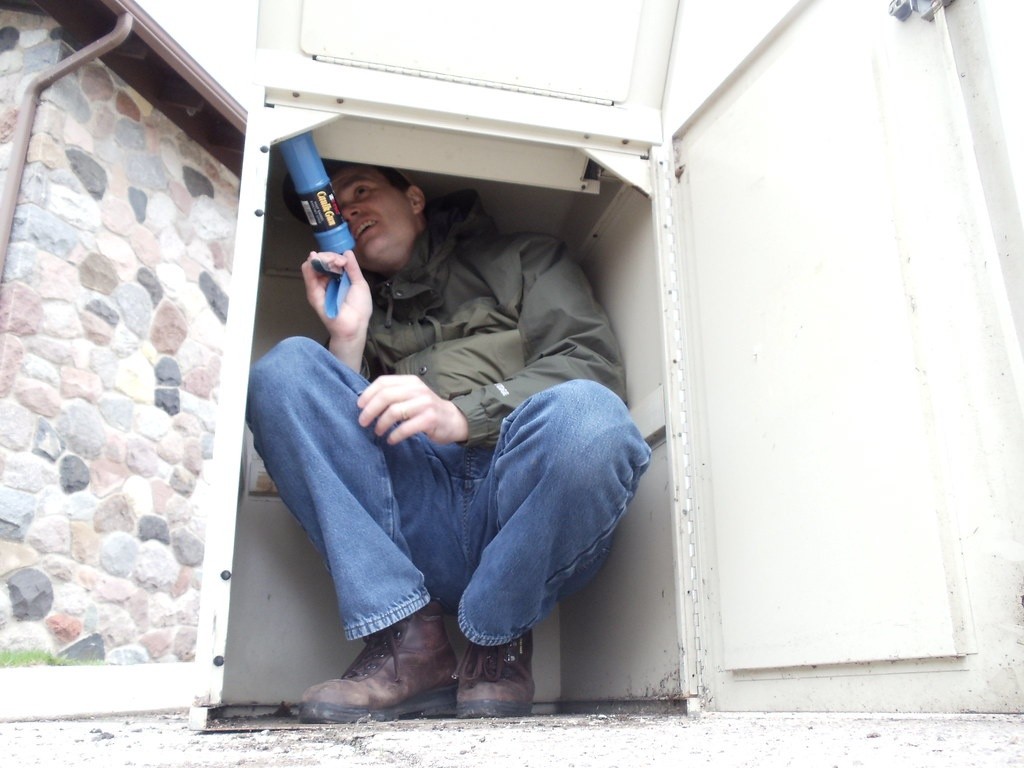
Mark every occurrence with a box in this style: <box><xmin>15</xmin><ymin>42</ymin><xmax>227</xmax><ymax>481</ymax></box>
<box><xmin>281</xmin><ymin>159</ymin><xmax>417</xmax><ymax>225</ymax></box>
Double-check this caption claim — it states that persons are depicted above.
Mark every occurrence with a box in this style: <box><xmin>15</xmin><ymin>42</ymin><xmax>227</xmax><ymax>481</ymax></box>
<box><xmin>245</xmin><ymin>158</ymin><xmax>650</xmax><ymax>721</ymax></box>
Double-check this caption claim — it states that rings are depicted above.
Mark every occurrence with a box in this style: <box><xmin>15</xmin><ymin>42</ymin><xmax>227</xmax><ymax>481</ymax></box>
<box><xmin>400</xmin><ymin>403</ymin><xmax>408</xmax><ymax>419</ymax></box>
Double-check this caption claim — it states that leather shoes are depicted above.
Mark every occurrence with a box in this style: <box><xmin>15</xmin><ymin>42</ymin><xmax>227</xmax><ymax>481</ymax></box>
<box><xmin>296</xmin><ymin>594</ymin><xmax>462</xmax><ymax>722</ymax></box>
<box><xmin>450</xmin><ymin>627</ymin><xmax>534</xmax><ymax>719</ymax></box>
